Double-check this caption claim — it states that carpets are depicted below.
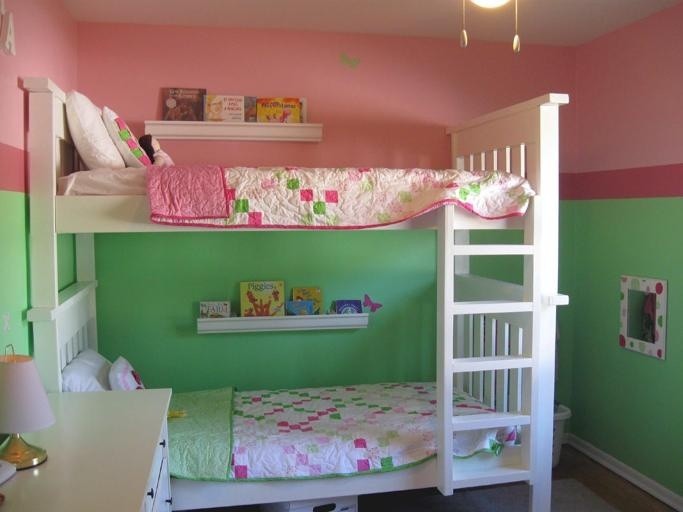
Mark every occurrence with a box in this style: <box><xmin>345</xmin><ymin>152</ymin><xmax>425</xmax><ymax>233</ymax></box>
<box><xmin>361</xmin><ymin>480</ymin><xmax>620</xmax><ymax>512</ymax></box>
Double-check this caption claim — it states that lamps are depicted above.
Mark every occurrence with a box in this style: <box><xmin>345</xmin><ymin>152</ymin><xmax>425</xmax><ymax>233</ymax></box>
<box><xmin>1</xmin><ymin>350</ymin><xmax>58</xmax><ymax>469</ymax></box>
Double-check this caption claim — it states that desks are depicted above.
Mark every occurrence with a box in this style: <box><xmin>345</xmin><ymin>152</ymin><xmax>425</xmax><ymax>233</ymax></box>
<box><xmin>1</xmin><ymin>387</ymin><xmax>178</xmax><ymax>511</ymax></box>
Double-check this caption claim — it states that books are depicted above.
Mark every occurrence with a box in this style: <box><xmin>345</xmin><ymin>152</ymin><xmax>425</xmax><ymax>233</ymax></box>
<box><xmin>336</xmin><ymin>299</ymin><xmax>362</xmax><ymax>315</ymax></box>
<box><xmin>240</xmin><ymin>281</ymin><xmax>286</xmax><ymax>317</ymax></box>
<box><xmin>286</xmin><ymin>300</ymin><xmax>313</xmax><ymax>316</ymax></box>
<box><xmin>199</xmin><ymin>299</ymin><xmax>231</xmax><ymax>318</ymax></box>
<box><xmin>293</xmin><ymin>288</ymin><xmax>323</xmax><ymax>315</ymax></box>
<box><xmin>161</xmin><ymin>87</ymin><xmax>308</xmax><ymax>124</ymax></box>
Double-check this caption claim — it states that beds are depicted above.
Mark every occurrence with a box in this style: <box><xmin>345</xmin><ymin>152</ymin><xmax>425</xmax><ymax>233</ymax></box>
<box><xmin>20</xmin><ymin>76</ymin><xmax>572</xmax><ymax>512</ymax></box>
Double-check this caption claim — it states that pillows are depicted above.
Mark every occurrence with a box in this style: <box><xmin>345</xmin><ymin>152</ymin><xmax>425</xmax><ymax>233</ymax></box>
<box><xmin>64</xmin><ymin>87</ymin><xmax>154</xmax><ymax>171</ymax></box>
<box><xmin>60</xmin><ymin>346</ymin><xmax>148</xmax><ymax>394</ymax></box>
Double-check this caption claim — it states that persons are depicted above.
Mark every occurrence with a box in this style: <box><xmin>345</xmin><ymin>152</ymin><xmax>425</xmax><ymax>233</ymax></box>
<box><xmin>139</xmin><ymin>134</ymin><xmax>176</xmax><ymax>166</ymax></box>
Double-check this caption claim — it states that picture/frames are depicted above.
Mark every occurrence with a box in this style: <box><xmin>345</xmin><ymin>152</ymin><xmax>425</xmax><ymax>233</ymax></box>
<box><xmin>619</xmin><ymin>274</ymin><xmax>668</xmax><ymax>360</ymax></box>
